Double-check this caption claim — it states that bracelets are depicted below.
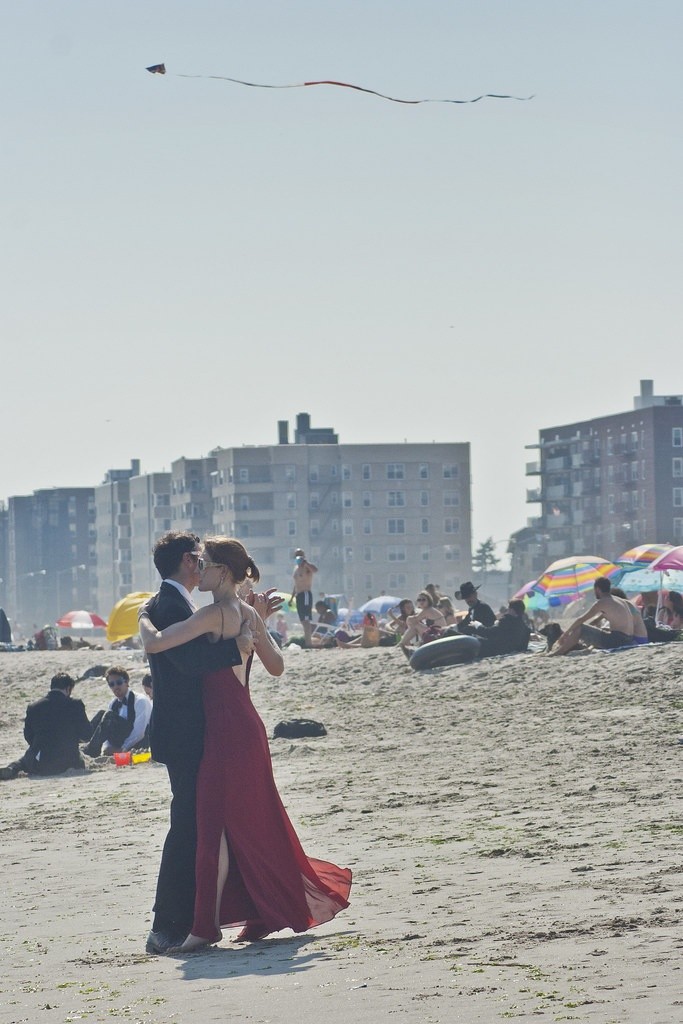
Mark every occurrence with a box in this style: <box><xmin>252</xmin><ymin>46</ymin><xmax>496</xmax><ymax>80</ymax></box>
<box><xmin>138</xmin><ymin>611</ymin><xmax>149</xmax><ymax>621</ymax></box>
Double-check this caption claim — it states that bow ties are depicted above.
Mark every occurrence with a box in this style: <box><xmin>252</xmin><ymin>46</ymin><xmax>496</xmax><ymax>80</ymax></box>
<box><xmin>115</xmin><ymin>696</ymin><xmax>128</xmax><ymax>709</ymax></box>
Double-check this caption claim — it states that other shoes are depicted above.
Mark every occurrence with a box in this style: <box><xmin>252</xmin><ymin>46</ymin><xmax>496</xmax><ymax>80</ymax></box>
<box><xmin>0</xmin><ymin>766</ymin><xmax>14</xmax><ymax>780</ymax></box>
<box><xmin>80</xmin><ymin>742</ymin><xmax>101</xmax><ymax>758</ymax></box>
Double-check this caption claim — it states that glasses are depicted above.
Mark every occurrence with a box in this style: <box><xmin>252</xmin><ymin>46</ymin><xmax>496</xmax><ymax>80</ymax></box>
<box><xmin>417</xmin><ymin>598</ymin><xmax>428</xmax><ymax>601</ymax></box>
<box><xmin>108</xmin><ymin>678</ymin><xmax>127</xmax><ymax>688</ymax></box>
<box><xmin>197</xmin><ymin>558</ymin><xmax>225</xmax><ymax>571</ymax></box>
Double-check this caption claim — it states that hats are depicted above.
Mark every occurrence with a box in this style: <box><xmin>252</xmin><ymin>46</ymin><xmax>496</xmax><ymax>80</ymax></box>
<box><xmin>454</xmin><ymin>581</ymin><xmax>482</xmax><ymax>600</ymax></box>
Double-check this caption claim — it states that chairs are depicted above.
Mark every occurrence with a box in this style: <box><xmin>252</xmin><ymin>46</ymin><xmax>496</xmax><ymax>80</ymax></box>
<box><xmin>311</xmin><ymin>597</ymin><xmax>354</xmax><ymax>648</ymax></box>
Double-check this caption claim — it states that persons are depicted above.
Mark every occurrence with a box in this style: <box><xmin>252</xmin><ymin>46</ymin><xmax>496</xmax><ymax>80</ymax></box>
<box><xmin>288</xmin><ymin>548</ymin><xmax>318</xmax><ymax>647</ymax></box>
<box><xmin>316</xmin><ymin>601</ymin><xmax>336</xmax><ymax>625</ymax></box>
<box><xmin>137</xmin><ymin>531</ymin><xmax>353</xmax><ymax>954</ymax></box>
<box><xmin>276</xmin><ymin>613</ymin><xmax>288</xmax><ymax>644</ymax></box>
<box><xmin>83</xmin><ymin>666</ymin><xmax>154</xmax><ymax>757</ymax></box>
<box><xmin>335</xmin><ymin>578</ymin><xmax>683</xmax><ymax>654</ymax></box>
<box><xmin>0</xmin><ymin>672</ymin><xmax>93</xmax><ymax>780</ymax></box>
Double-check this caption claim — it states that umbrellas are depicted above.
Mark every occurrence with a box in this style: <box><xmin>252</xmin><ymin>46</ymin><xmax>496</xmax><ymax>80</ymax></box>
<box><xmin>106</xmin><ymin>591</ymin><xmax>155</xmax><ymax>642</ymax></box>
<box><xmin>358</xmin><ymin>595</ymin><xmax>405</xmax><ymax>614</ymax></box>
<box><xmin>57</xmin><ymin>609</ymin><xmax>106</xmax><ymax>628</ymax></box>
<box><xmin>269</xmin><ymin>592</ymin><xmax>297</xmax><ymax>612</ymax></box>
<box><xmin>337</xmin><ymin>608</ymin><xmax>363</xmax><ymax>624</ymax></box>
<box><xmin>507</xmin><ymin>541</ymin><xmax>683</xmax><ymax>609</ymax></box>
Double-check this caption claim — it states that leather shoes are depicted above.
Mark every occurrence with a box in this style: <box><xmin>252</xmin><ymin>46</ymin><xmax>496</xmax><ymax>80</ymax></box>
<box><xmin>145</xmin><ymin>929</ymin><xmax>187</xmax><ymax>954</ymax></box>
